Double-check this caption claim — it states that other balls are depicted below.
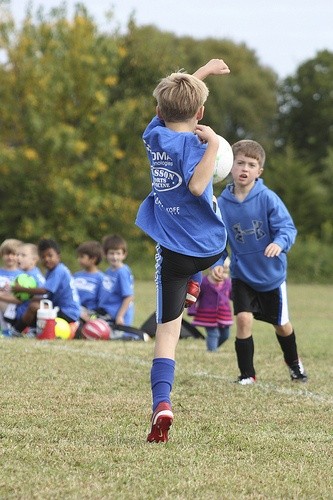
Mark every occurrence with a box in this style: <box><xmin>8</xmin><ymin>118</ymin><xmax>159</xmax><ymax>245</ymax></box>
<box><xmin>54</xmin><ymin>318</ymin><xmax>71</xmax><ymax>340</ymax></box>
<box><xmin>80</xmin><ymin>317</ymin><xmax>111</xmax><ymax>341</ymax></box>
<box><xmin>12</xmin><ymin>272</ymin><xmax>37</xmax><ymax>302</ymax></box>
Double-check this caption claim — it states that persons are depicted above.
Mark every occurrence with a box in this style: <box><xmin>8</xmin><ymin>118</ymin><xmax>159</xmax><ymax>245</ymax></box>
<box><xmin>208</xmin><ymin>140</ymin><xmax>307</xmax><ymax>384</ymax></box>
<box><xmin>0</xmin><ymin>235</ymin><xmax>138</xmax><ymax>341</ymax></box>
<box><xmin>187</xmin><ymin>258</ymin><xmax>232</xmax><ymax>353</ymax></box>
<box><xmin>134</xmin><ymin>58</ymin><xmax>231</xmax><ymax>442</ymax></box>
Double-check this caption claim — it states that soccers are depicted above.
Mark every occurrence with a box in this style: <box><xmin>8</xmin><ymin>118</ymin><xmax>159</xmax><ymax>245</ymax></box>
<box><xmin>196</xmin><ymin>133</ymin><xmax>234</xmax><ymax>186</ymax></box>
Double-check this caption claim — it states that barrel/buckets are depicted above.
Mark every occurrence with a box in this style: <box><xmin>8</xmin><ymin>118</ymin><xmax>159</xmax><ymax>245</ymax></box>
<box><xmin>36</xmin><ymin>299</ymin><xmax>59</xmax><ymax>334</ymax></box>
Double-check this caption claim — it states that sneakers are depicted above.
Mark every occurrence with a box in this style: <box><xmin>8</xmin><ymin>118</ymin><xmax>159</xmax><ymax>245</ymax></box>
<box><xmin>286</xmin><ymin>359</ymin><xmax>307</xmax><ymax>383</ymax></box>
<box><xmin>184</xmin><ymin>279</ymin><xmax>200</xmax><ymax>308</ymax></box>
<box><xmin>147</xmin><ymin>402</ymin><xmax>174</xmax><ymax>441</ymax></box>
<box><xmin>239</xmin><ymin>374</ymin><xmax>255</xmax><ymax>384</ymax></box>
